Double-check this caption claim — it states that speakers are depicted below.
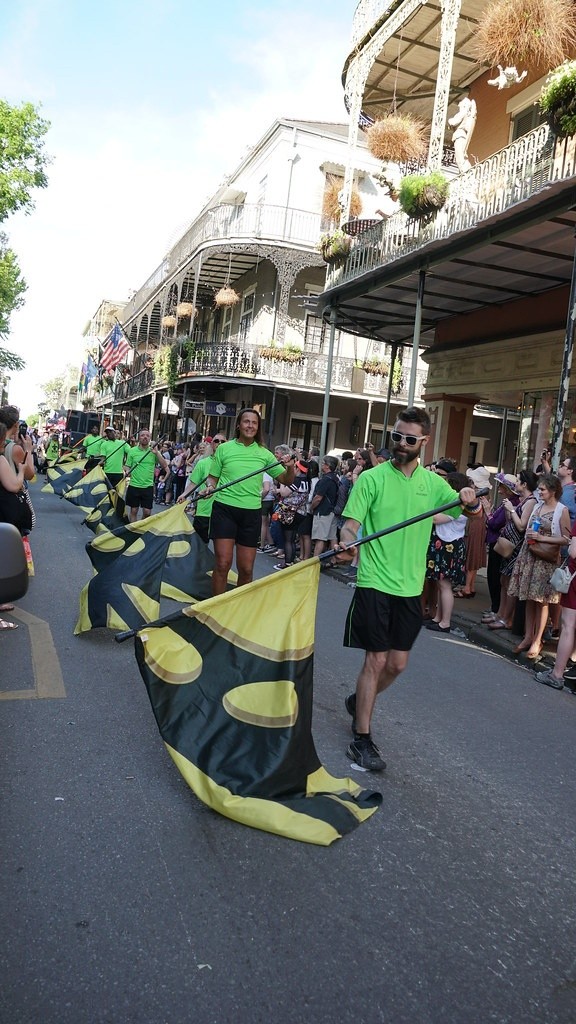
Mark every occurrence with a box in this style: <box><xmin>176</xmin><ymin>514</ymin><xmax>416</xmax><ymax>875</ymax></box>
<box><xmin>64</xmin><ymin>410</ymin><xmax>100</xmax><ymax>448</ymax></box>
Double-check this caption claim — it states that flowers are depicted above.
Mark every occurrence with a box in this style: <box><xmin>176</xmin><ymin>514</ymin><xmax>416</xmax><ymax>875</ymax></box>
<box><xmin>533</xmin><ymin>57</ymin><xmax>576</xmax><ymax>134</ymax></box>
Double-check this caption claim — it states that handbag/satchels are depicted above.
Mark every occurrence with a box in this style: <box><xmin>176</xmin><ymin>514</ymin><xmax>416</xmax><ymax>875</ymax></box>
<box><xmin>550</xmin><ymin>566</ymin><xmax>572</xmax><ymax>593</ymax></box>
<box><xmin>272</xmin><ymin>506</ymin><xmax>297</xmax><ymax>525</ymax></box>
<box><xmin>528</xmin><ymin>539</ymin><xmax>560</xmax><ymax>565</ymax></box>
<box><xmin>493</xmin><ymin>537</ymin><xmax>515</xmax><ymax>558</ymax></box>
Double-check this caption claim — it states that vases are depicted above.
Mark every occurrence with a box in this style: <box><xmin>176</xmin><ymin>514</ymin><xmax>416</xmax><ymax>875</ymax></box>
<box><xmin>547</xmin><ymin>91</ymin><xmax>576</xmax><ymax>138</ymax></box>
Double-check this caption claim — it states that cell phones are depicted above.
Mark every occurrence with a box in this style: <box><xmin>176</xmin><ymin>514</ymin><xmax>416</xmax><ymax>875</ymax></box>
<box><xmin>22</xmin><ymin>451</ymin><xmax>28</xmax><ymax>464</ymax></box>
<box><xmin>365</xmin><ymin>442</ymin><xmax>370</xmax><ymax>449</ymax></box>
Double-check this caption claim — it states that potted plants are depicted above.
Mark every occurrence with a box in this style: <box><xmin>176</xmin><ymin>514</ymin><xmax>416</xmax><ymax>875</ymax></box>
<box><xmin>316</xmin><ymin>230</ymin><xmax>351</xmax><ymax>264</ymax></box>
<box><xmin>400</xmin><ymin>171</ymin><xmax>448</xmax><ymax>218</ymax></box>
<box><xmin>166</xmin><ymin>337</ymin><xmax>195</xmax><ymax>399</ymax></box>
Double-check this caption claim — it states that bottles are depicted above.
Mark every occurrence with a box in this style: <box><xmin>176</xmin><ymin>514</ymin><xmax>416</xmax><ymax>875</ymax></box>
<box><xmin>531</xmin><ymin>517</ymin><xmax>541</xmax><ymax>531</ymax></box>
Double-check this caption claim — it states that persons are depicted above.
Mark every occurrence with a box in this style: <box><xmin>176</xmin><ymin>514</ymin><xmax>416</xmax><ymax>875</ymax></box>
<box><xmin>331</xmin><ymin>407</ymin><xmax>482</xmax><ymax>771</ymax></box>
<box><xmin>262</xmin><ymin>444</ymin><xmax>391</xmax><ymax>573</ymax></box>
<box><xmin>448</xmin><ymin>97</ymin><xmax>477</xmax><ymax>173</ymax></box>
<box><xmin>424</xmin><ymin>449</ymin><xmax>576</xmax><ymax>690</ymax></box>
<box><xmin>198</xmin><ymin>409</ymin><xmax>295</xmax><ymax>596</ymax></box>
<box><xmin>150</xmin><ymin>432</ymin><xmax>213</xmax><ymax>506</ymax></box>
<box><xmin>0</xmin><ymin>406</ymin><xmax>60</xmax><ymax>537</ymax></box>
<box><xmin>98</xmin><ymin>426</ymin><xmax>131</xmax><ymax>487</ymax></box>
<box><xmin>80</xmin><ymin>424</ymin><xmax>106</xmax><ymax>474</ymax></box>
<box><xmin>123</xmin><ymin>428</ymin><xmax>168</xmax><ymax>522</ymax></box>
<box><xmin>176</xmin><ymin>433</ymin><xmax>228</xmax><ymax>547</ymax></box>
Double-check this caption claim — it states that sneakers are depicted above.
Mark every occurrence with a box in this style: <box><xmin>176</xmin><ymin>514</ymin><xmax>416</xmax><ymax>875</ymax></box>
<box><xmin>345</xmin><ymin>693</ymin><xmax>371</xmax><ymax>737</ymax></box>
<box><xmin>345</xmin><ymin>738</ymin><xmax>386</xmax><ymax>770</ymax></box>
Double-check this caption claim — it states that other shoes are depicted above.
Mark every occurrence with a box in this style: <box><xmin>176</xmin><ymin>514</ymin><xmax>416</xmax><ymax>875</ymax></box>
<box><xmin>153</xmin><ymin>495</ymin><xmax>196</xmax><ymax>518</ymax></box>
<box><xmin>421</xmin><ymin>605</ymin><xmax>576</xmax><ymax>690</ymax></box>
<box><xmin>256</xmin><ymin>536</ymin><xmax>358</xmax><ymax>578</ymax></box>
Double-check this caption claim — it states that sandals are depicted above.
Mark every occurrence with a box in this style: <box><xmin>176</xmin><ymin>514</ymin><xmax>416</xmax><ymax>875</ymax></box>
<box><xmin>0</xmin><ymin>618</ymin><xmax>19</xmax><ymax>630</ymax></box>
<box><xmin>0</xmin><ymin>602</ymin><xmax>14</xmax><ymax>611</ymax></box>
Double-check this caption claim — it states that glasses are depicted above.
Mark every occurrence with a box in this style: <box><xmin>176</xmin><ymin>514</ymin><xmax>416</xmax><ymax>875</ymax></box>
<box><xmin>358</xmin><ymin>457</ymin><xmax>363</xmax><ymax>460</ymax></box>
<box><xmin>434</xmin><ymin>472</ymin><xmax>448</xmax><ymax>476</ymax></box>
<box><xmin>390</xmin><ymin>429</ymin><xmax>425</xmax><ymax>446</ymax></box>
<box><xmin>539</xmin><ymin>488</ymin><xmax>546</xmax><ymax>491</ymax></box>
<box><xmin>560</xmin><ymin>462</ymin><xmax>568</xmax><ymax>468</ymax></box>
<box><xmin>213</xmin><ymin>439</ymin><xmax>226</xmax><ymax>444</ymax></box>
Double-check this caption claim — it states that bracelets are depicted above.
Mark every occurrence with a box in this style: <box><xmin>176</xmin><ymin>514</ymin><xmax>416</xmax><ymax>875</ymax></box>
<box><xmin>510</xmin><ymin>510</ymin><xmax>515</xmax><ymax>513</ymax></box>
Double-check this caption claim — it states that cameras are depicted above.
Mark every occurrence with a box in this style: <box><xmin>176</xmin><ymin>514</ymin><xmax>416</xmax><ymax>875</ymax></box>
<box><xmin>18</xmin><ymin>423</ymin><xmax>27</xmax><ymax>439</ymax></box>
<box><xmin>542</xmin><ymin>451</ymin><xmax>547</xmax><ymax>461</ymax></box>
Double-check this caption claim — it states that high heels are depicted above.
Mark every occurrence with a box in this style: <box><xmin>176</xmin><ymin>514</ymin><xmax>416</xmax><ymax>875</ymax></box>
<box><xmin>452</xmin><ymin>589</ymin><xmax>476</xmax><ymax>599</ymax></box>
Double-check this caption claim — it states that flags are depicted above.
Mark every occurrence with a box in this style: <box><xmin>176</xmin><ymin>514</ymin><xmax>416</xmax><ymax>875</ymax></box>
<box><xmin>136</xmin><ymin>563</ymin><xmax>384</xmax><ymax>847</ymax></box>
<box><xmin>100</xmin><ymin>322</ymin><xmax>130</xmax><ymax>373</ymax></box>
<box><xmin>74</xmin><ymin>499</ymin><xmax>238</xmax><ymax>636</ymax></box>
<box><xmin>41</xmin><ymin>451</ymin><xmax>130</xmax><ymax>534</ymax></box>
<box><xmin>78</xmin><ymin>346</ymin><xmax>106</xmax><ymax>393</ymax></box>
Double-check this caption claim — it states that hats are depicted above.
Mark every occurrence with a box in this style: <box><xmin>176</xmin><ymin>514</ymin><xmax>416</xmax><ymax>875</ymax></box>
<box><xmin>374</xmin><ymin>447</ymin><xmax>390</xmax><ymax>460</ymax></box>
<box><xmin>435</xmin><ymin>462</ymin><xmax>457</xmax><ymax>474</ymax></box>
<box><xmin>175</xmin><ymin>444</ymin><xmax>182</xmax><ymax>449</ymax></box>
<box><xmin>466</xmin><ymin>467</ymin><xmax>492</xmax><ymax>489</ymax></box>
<box><xmin>493</xmin><ymin>473</ymin><xmax>524</xmax><ymax>497</ymax></box>
<box><xmin>104</xmin><ymin>426</ymin><xmax>116</xmax><ymax>431</ymax></box>
<box><xmin>204</xmin><ymin>436</ymin><xmax>212</xmax><ymax>443</ymax></box>
